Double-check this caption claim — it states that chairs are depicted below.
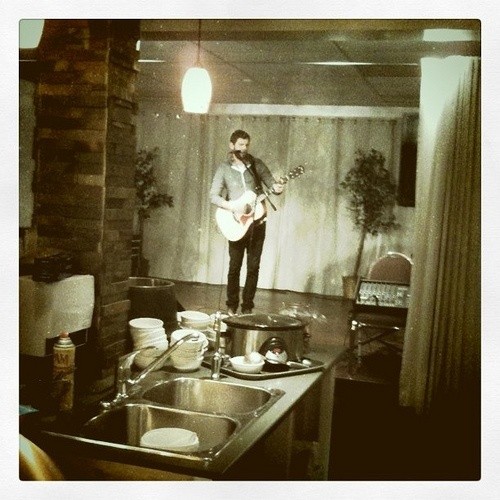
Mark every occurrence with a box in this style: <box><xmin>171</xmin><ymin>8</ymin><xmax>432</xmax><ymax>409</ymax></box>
<box><xmin>347</xmin><ymin>252</ymin><xmax>413</xmax><ymax>375</ymax></box>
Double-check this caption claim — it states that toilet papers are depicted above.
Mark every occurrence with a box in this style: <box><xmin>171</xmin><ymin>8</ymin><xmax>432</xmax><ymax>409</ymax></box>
<box><xmin>18</xmin><ymin>274</ymin><xmax>95</xmax><ymax>357</ymax></box>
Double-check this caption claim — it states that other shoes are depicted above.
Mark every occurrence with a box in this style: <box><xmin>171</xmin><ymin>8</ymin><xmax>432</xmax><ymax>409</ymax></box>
<box><xmin>242</xmin><ymin>309</ymin><xmax>252</xmax><ymax>313</ymax></box>
<box><xmin>227</xmin><ymin>308</ymin><xmax>237</xmax><ymax>314</ymax></box>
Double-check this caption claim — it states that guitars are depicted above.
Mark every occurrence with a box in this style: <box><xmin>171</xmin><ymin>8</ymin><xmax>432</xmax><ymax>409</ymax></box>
<box><xmin>216</xmin><ymin>165</ymin><xmax>305</xmax><ymax>242</ymax></box>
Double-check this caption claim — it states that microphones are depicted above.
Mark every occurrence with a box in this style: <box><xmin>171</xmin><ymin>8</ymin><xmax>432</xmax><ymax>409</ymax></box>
<box><xmin>241</xmin><ymin>153</ymin><xmax>254</xmax><ymax>162</ymax></box>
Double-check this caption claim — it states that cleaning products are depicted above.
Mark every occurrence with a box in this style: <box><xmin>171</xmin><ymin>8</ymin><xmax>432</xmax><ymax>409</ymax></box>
<box><xmin>52</xmin><ymin>332</ymin><xmax>76</xmax><ymax>411</ymax></box>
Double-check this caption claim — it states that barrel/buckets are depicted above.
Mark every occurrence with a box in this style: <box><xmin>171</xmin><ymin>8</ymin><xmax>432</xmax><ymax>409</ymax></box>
<box><xmin>125</xmin><ymin>277</ymin><xmax>175</xmax><ymax>331</ymax></box>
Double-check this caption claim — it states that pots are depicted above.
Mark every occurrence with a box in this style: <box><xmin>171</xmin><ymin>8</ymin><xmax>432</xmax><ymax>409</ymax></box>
<box><xmin>224</xmin><ymin>314</ymin><xmax>308</xmax><ymax>362</ymax></box>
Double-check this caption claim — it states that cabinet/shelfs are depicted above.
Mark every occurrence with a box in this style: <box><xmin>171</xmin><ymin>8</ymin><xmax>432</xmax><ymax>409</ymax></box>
<box><xmin>20</xmin><ymin>275</ymin><xmax>95</xmax><ymax>410</ymax></box>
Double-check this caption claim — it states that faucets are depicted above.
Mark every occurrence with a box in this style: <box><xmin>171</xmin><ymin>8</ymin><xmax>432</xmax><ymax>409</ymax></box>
<box><xmin>129</xmin><ymin>333</ymin><xmax>199</xmax><ymax>387</ymax></box>
<box><xmin>213</xmin><ymin>317</ymin><xmax>223</xmax><ymax>354</ymax></box>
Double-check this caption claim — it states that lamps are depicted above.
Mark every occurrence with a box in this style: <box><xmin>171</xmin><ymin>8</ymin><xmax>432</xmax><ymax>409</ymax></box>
<box><xmin>181</xmin><ymin>21</ymin><xmax>212</xmax><ymax>114</ymax></box>
<box><xmin>19</xmin><ymin>19</ymin><xmax>45</xmax><ymax>49</ymax></box>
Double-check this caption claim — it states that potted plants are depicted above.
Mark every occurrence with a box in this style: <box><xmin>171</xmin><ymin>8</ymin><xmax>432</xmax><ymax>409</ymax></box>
<box><xmin>341</xmin><ymin>149</ymin><xmax>401</xmax><ymax>299</ymax></box>
<box><xmin>132</xmin><ymin>146</ymin><xmax>174</xmax><ymax>276</ymax></box>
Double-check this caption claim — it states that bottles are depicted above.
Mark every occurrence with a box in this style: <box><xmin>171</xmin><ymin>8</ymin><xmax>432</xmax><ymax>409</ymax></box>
<box><xmin>51</xmin><ymin>332</ymin><xmax>74</xmax><ymax>409</ymax></box>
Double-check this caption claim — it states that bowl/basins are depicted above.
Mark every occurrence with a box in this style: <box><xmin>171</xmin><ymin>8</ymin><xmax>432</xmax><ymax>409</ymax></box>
<box><xmin>128</xmin><ymin>318</ymin><xmax>168</xmax><ymax>371</ymax></box>
<box><xmin>230</xmin><ymin>356</ymin><xmax>265</xmax><ymax>374</ymax></box>
<box><xmin>140</xmin><ymin>428</ymin><xmax>199</xmax><ymax>455</ymax></box>
<box><xmin>179</xmin><ymin>311</ymin><xmax>211</xmax><ymax>336</ymax></box>
<box><xmin>170</xmin><ymin>329</ymin><xmax>209</xmax><ymax>371</ymax></box>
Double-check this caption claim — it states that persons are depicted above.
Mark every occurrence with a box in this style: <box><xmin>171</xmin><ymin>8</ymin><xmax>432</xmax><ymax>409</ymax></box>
<box><xmin>206</xmin><ymin>129</ymin><xmax>286</xmax><ymax>318</ymax></box>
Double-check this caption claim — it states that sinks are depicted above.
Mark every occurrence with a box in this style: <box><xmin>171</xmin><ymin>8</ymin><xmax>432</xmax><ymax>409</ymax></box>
<box><xmin>142</xmin><ymin>377</ymin><xmax>271</xmax><ymax>415</ymax></box>
<box><xmin>83</xmin><ymin>403</ymin><xmax>237</xmax><ymax>451</ymax></box>
